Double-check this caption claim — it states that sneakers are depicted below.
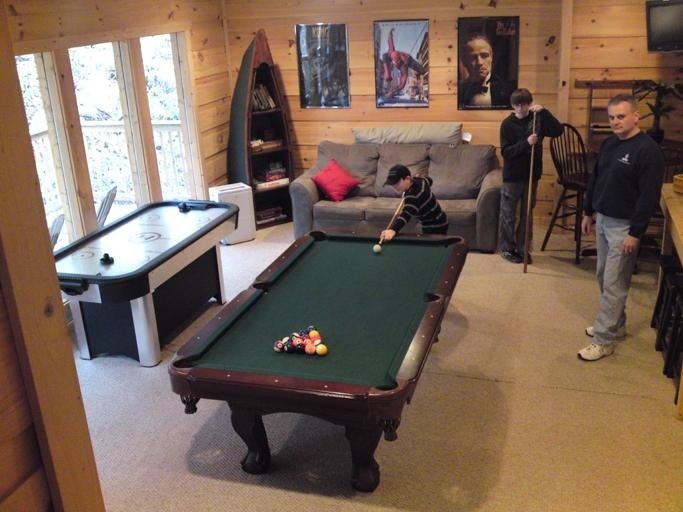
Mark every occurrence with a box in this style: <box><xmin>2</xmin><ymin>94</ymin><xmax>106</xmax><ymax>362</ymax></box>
<box><xmin>577</xmin><ymin>342</ymin><xmax>614</xmax><ymax>361</ymax></box>
<box><xmin>584</xmin><ymin>326</ymin><xmax>628</xmax><ymax>341</ymax></box>
<box><xmin>500</xmin><ymin>249</ymin><xmax>532</xmax><ymax>265</ymax></box>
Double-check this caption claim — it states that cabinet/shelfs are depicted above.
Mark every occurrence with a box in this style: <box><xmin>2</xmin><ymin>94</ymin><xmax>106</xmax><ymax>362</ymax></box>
<box><xmin>227</xmin><ymin>27</ymin><xmax>294</xmax><ymax>231</ymax></box>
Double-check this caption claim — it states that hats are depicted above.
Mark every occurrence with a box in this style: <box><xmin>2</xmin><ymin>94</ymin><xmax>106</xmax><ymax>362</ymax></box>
<box><xmin>382</xmin><ymin>165</ymin><xmax>411</xmax><ymax>188</ymax></box>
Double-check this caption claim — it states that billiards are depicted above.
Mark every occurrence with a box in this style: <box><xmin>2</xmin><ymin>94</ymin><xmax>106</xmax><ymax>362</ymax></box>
<box><xmin>273</xmin><ymin>324</ymin><xmax>327</xmax><ymax>355</ymax></box>
<box><xmin>373</xmin><ymin>244</ymin><xmax>382</xmax><ymax>253</ymax></box>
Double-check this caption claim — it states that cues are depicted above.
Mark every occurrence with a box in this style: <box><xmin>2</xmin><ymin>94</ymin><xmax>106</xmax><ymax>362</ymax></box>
<box><xmin>379</xmin><ymin>197</ymin><xmax>405</xmax><ymax>243</ymax></box>
<box><xmin>524</xmin><ymin>111</ymin><xmax>536</xmax><ymax>273</ymax></box>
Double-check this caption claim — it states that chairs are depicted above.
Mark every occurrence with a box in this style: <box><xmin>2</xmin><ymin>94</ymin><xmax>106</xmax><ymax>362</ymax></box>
<box><xmin>632</xmin><ymin>146</ymin><xmax>683</xmax><ymax>274</ymax></box>
<box><xmin>47</xmin><ymin>212</ymin><xmax>65</xmax><ymax>251</ymax></box>
<box><xmin>540</xmin><ymin>122</ymin><xmax>593</xmax><ymax>265</ymax></box>
<box><xmin>649</xmin><ymin>147</ymin><xmax>683</xmax><ymax>218</ymax></box>
<box><xmin>96</xmin><ymin>185</ymin><xmax>117</xmax><ymax>227</ymax></box>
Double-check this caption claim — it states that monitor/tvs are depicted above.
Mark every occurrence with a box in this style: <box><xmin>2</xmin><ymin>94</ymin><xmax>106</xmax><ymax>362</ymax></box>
<box><xmin>646</xmin><ymin>0</ymin><xmax>683</xmax><ymax>51</ymax></box>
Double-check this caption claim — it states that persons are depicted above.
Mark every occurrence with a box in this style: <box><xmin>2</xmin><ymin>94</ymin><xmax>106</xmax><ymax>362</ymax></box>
<box><xmin>376</xmin><ymin>163</ymin><xmax>449</xmax><ymax>244</ymax></box>
<box><xmin>458</xmin><ymin>34</ymin><xmax>510</xmax><ymax>105</ymax></box>
<box><xmin>576</xmin><ymin>94</ymin><xmax>665</xmax><ymax>362</ymax></box>
<box><xmin>496</xmin><ymin>87</ymin><xmax>564</xmax><ymax>264</ymax></box>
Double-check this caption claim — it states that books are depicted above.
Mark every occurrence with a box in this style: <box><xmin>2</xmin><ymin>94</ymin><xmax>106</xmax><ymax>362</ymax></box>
<box><xmin>250</xmin><ymin>83</ymin><xmax>277</xmax><ymax>112</ymax></box>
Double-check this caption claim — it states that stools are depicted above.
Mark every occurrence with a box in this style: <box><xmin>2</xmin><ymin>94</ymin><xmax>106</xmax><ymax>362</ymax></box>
<box><xmin>649</xmin><ymin>253</ymin><xmax>683</xmax><ymax>378</ymax></box>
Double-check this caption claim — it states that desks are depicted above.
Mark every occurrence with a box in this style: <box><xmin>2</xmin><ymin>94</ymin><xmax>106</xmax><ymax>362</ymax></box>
<box><xmin>167</xmin><ymin>229</ymin><xmax>469</xmax><ymax>493</ymax></box>
<box><xmin>53</xmin><ymin>199</ymin><xmax>240</xmax><ymax>368</ymax></box>
<box><xmin>654</xmin><ymin>182</ymin><xmax>683</xmax><ymax>420</ymax></box>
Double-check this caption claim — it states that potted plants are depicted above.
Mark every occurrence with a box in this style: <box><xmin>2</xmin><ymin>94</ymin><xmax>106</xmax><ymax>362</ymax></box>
<box><xmin>631</xmin><ymin>76</ymin><xmax>682</xmax><ymax>143</ymax></box>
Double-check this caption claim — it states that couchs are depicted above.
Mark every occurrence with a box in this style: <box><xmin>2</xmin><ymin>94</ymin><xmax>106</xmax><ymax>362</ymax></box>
<box><xmin>289</xmin><ymin>155</ymin><xmax>504</xmax><ymax>254</ymax></box>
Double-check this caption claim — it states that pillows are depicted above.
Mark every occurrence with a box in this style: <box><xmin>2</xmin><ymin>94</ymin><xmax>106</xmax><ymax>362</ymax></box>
<box><xmin>374</xmin><ymin>143</ymin><xmax>431</xmax><ymax>198</ymax></box>
<box><xmin>428</xmin><ymin>142</ymin><xmax>497</xmax><ymax>199</ymax></box>
<box><xmin>350</xmin><ymin>122</ymin><xmax>463</xmax><ymax>145</ymax></box>
<box><xmin>311</xmin><ymin>158</ymin><xmax>361</xmax><ymax>204</ymax></box>
<box><xmin>316</xmin><ymin>139</ymin><xmax>379</xmax><ymax>198</ymax></box>
